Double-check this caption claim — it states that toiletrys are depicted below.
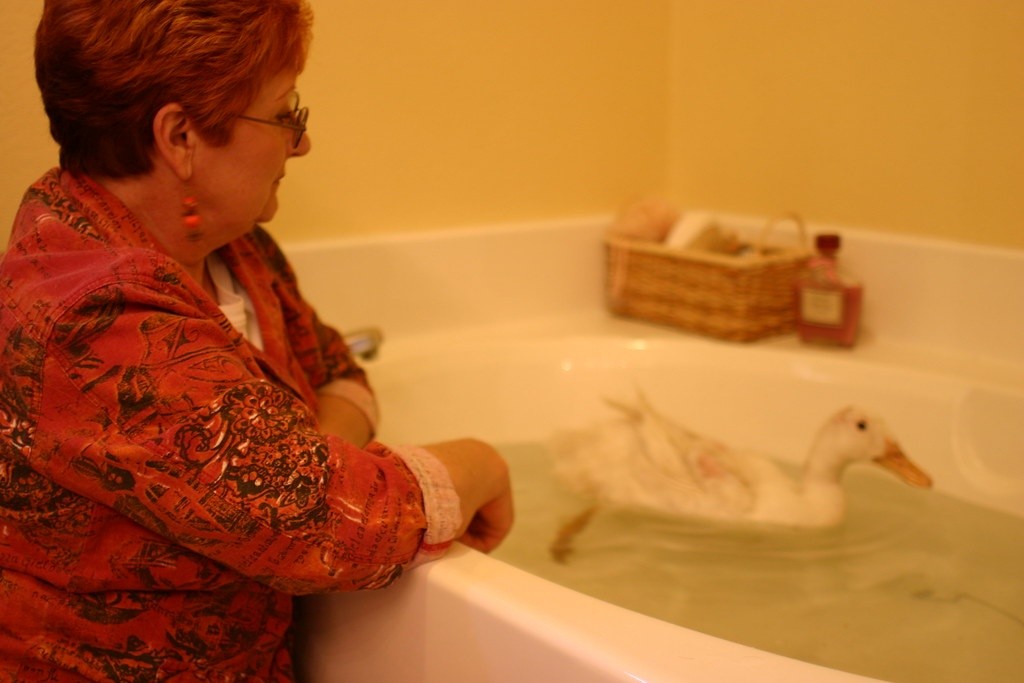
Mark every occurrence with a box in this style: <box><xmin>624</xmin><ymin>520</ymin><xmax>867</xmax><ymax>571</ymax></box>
<box><xmin>798</xmin><ymin>231</ymin><xmax>862</xmax><ymax>345</ymax></box>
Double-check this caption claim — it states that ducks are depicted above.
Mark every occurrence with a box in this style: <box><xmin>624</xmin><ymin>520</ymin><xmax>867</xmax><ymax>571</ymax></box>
<box><xmin>546</xmin><ymin>382</ymin><xmax>936</xmax><ymax>555</ymax></box>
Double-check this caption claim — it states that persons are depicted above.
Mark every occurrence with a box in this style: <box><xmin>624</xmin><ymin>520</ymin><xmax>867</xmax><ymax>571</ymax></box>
<box><xmin>0</xmin><ymin>0</ymin><xmax>514</xmax><ymax>682</ymax></box>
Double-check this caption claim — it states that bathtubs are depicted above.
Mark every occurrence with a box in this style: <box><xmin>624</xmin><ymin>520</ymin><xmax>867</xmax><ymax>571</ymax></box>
<box><xmin>286</xmin><ymin>295</ymin><xmax>1022</xmax><ymax>682</ymax></box>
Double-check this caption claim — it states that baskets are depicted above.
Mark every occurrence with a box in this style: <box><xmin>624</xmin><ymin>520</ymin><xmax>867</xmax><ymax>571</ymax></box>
<box><xmin>604</xmin><ymin>211</ymin><xmax>813</xmax><ymax>343</ymax></box>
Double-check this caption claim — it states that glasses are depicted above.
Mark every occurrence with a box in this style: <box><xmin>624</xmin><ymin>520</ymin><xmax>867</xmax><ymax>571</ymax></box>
<box><xmin>231</xmin><ymin>91</ymin><xmax>310</xmax><ymax>149</ymax></box>
